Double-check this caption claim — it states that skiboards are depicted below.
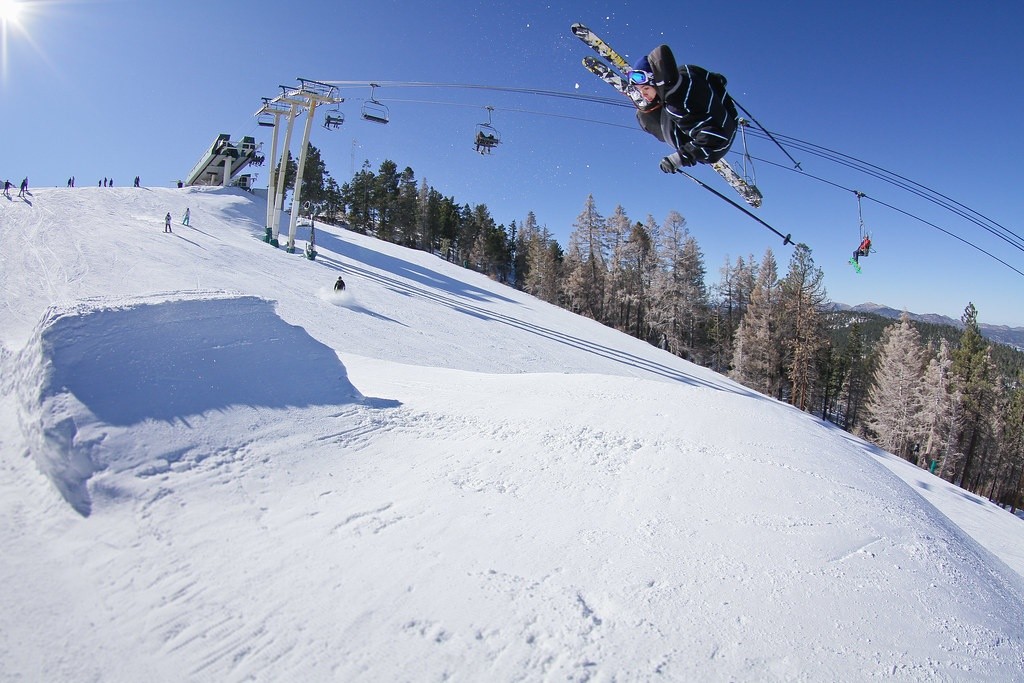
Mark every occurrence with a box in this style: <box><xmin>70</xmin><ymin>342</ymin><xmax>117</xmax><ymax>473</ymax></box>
<box><xmin>570</xmin><ymin>23</ymin><xmax>764</xmax><ymax>208</ymax></box>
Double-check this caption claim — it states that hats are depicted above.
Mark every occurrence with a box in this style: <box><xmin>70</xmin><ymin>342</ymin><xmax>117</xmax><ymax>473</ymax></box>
<box><xmin>338</xmin><ymin>276</ymin><xmax>341</xmax><ymax>279</ymax></box>
<box><xmin>629</xmin><ymin>55</ymin><xmax>655</xmax><ymax>86</ymax></box>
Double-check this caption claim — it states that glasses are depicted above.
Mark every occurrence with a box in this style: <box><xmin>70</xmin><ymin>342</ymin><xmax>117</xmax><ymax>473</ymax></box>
<box><xmin>629</xmin><ymin>69</ymin><xmax>653</xmax><ymax>84</ymax></box>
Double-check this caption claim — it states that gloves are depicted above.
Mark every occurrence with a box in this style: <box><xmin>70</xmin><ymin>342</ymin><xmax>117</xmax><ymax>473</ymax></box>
<box><xmin>658</xmin><ymin>151</ymin><xmax>683</xmax><ymax>174</ymax></box>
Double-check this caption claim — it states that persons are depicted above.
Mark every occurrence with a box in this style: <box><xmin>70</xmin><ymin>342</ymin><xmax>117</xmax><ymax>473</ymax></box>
<box><xmin>24</xmin><ymin>176</ymin><xmax>28</xmax><ymax>191</ymax></box>
<box><xmin>67</xmin><ymin>177</ymin><xmax>71</xmax><ymax>187</ymax></box>
<box><xmin>134</xmin><ymin>176</ymin><xmax>140</xmax><ymax>186</ymax></box>
<box><xmin>2</xmin><ymin>180</ymin><xmax>12</xmax><ymax>194</ymax></box>
<box><xmin>109</xmin><ymin>178</ymin><xmax>113</xmax><ymax>187</ymax></box>
<box><xmin>98</xmin><ymin>178</ymin><xmax>102</xmax><ymax>187</ymax></box>
<box><xmin>165</xmin><ymin>211</ymin><xmax>172</xmax><ymax>232</ymax></box>
<box><xmin>325</xmin><ymin>114</ymin><xmax>341</xmax><ymax>129</ymax></box>
<box><xmin>850</xmin><ymin>233</ymin><xmax>870</xmax><ymax>265</ymax></box>
<box><xmin>627</xmin><ymin>45</ymin><xmax>739</xmax><ymax>175</ymax></box>
<box><xmin>333</xmin><ymin>276</ymin><xmax>345</xmax><ymax>290</ymax></box>
<box><xmin>17</xmin><ymin>180</ymin><xmax>27</xmax><ymax>197</ymax></box>
<box><xmin>476</xmin><ymin>130</ymin><xmax>496</xmax><ymax>156</ymax></box>
<box><xmin>177</xmin><ymin>179</ymin><xmax>183</xmax><ymax>188</ymax></box>
<box><xmin>71</xmin><ymin>176</ymin><xmax>75</xmax><ymax>187</ymax></box>
<box><xmin>103</xmin><ymin>176</ymin><xmax>107</xmax><ymax>187</ymax></box>
<box><xmin>182</xmin><ymin>207</ymin><xmax>190</xmax><ymax>226</ymax></box>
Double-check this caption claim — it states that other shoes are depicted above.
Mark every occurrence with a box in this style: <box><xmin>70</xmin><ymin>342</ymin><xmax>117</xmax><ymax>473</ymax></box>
<box><xmin>169</xmin><ymin>228</ymin><xmax>171</xmax><ymax>232</ymax></box>
<box><xmin>165</xmin><ymin>229</ymin><xmax>167</xmax><ymax>232</ymax></box>
<box><xmin>333</xmin><ymin>125</ymin><xmax>335</xmax><ymax>127</ymax></box>
<box><xmin>327</xmin><ymin>124</ymin><xmax>329</xmax><ymax>127</ymax></box>
<box><xmin>324</xmin><ymin>124</ymin><xmax>326</xmax><ymax>127</ymax></box>
